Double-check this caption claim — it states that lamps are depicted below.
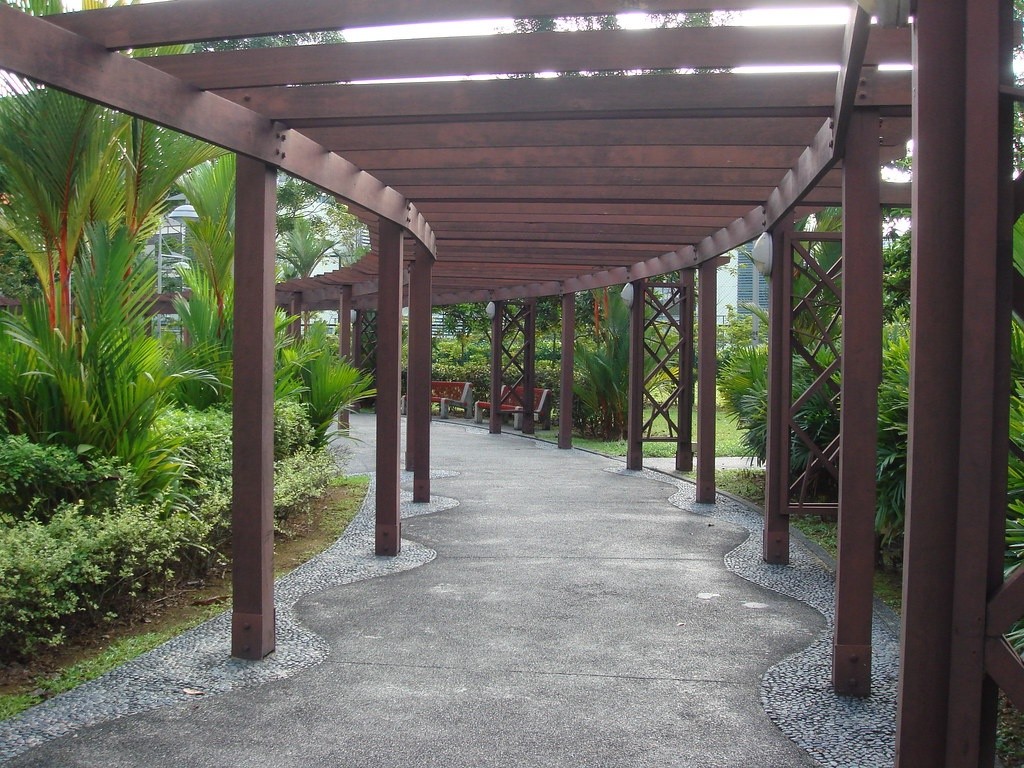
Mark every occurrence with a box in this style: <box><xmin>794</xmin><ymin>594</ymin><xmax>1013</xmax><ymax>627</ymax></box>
<box><xmin>485</xmin><ymin>301</ymin><xmax>495</xmax><ymax>320</ymax></box>
<box><xmin>350</xmin><ymin>310</ymin><xmax>356</xmax><ymax>324</ymax></box>
<box><xmin>620</xmin><ymin>282</ymin><xmax>633</xmax><ymax>308</ymax></box>
<box><xmin>751</xmin><ymin>231</ymin><xmax>773</xmax><ymax>276</ymax></box>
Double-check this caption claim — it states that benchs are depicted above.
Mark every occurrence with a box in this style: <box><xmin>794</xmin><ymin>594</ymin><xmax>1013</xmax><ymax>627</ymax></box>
<box><xmin>401</xmin><ymin>382</ymin><xmax>474</xmax><ymax>419</ymax></box>
<box><xmin>474</xmin><ymin>385</ymin><xmax>552</xmax><ymax>431</ymax></box>
